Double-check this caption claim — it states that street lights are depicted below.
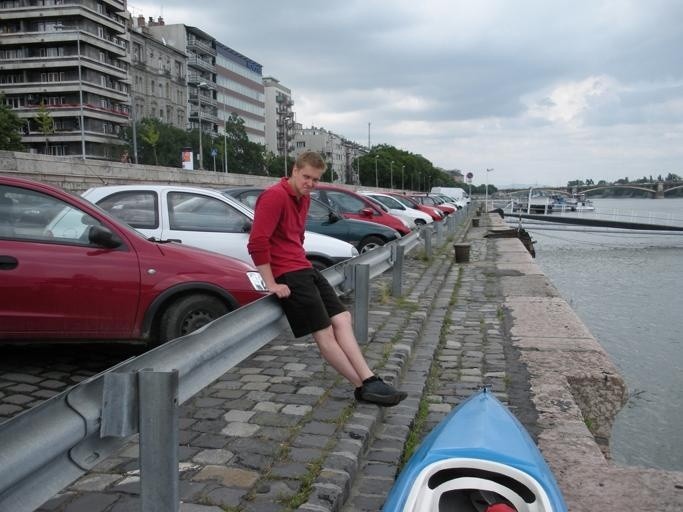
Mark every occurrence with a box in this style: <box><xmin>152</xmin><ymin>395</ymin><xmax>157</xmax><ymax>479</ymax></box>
<box><xmin>366</xmin><ymin>122</ymin><xmax>372</xmax><ymax>151</ymax></box>
<box><xmin>194</xmin><ymin>81</ymin><xmax>206</xmax><ymax>169</ymax></box>
<box><xmin>528</xmin><ymin>183</ymin><xmax>537</xmax><ymax>216</ymax></box>
<box><xmin>52</xmin><ymin>21</ymin><xmax>86</xmax><ymax>159</ymax></box>
<box><xmin>485</xmin><ymin>168</ymin><xmax>495</xmax><ymax>214</ymax></box>
<box><xmin>329</xmin><ymin>134</ymin><xmax>449</xmax><ymax>194</ymax></box>
<box><xmin>283</xmin><ymin>117</ymin><xmax>290</xmax><ymax>177</ymax></box>
<box><xmin>126</xmin><ymin>24</ymin><xmax>166</xmax><ymax>164</ymax></box>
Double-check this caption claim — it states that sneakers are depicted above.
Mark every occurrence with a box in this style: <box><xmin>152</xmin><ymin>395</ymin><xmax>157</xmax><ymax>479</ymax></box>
<box><xmin>353</xmin><ymin>376</ymin><xmax>408</xmax><ymax>408</ymax></box>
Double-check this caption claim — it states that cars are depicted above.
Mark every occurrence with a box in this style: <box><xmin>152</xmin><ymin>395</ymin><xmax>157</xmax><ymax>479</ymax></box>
<box><xmin>170</xmin><ymin>189</ymin><xmax>401</xmax><ymax>261</ymax></box>
<box><xmin>19</xmin><ymin>190</ymin><xmax>64</xmax><ymax>226</ymax></box>
<box><xmin>0</xmin><ymin>177</ymin><xmax>273</xmax><ymax>353</ymax></box>
<box><xmin>45</xmin><ymin>183</ymin><xmax>359</xmax><ymax>305</ymax></box>
<box><xmin>306</xmin><ymin>186</ymin><xmax>471</xmax><ymax>236</ymax></box>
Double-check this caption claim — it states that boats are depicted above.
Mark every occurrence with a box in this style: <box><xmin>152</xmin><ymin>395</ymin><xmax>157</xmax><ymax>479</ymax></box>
<box><xmin>515</xmin><ymin>192</ymin><xmax>594</xmax><ymax>214</ymax></box>
<box><xmin>381</xmin><ymin>383</ymin><xmax>566</xmax><ymax>512</ymax></box>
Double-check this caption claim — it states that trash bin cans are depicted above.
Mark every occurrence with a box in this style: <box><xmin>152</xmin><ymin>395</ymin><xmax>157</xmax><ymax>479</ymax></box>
<box><xmin>180</xmin><ymin>147</ymin><xmax>193</xmax><ymax>170</ymax></box>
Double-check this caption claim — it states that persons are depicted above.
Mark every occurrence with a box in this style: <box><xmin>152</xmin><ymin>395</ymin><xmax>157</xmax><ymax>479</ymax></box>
<box><xmin>121</xmin><ymin>150</ymin><xmax>131</xmax><ymax>164</ymax></box>
<box><xmin>246</xmin><ymin>151</ymin><xmax>408</xmax><ymax>407</ymax></box>
